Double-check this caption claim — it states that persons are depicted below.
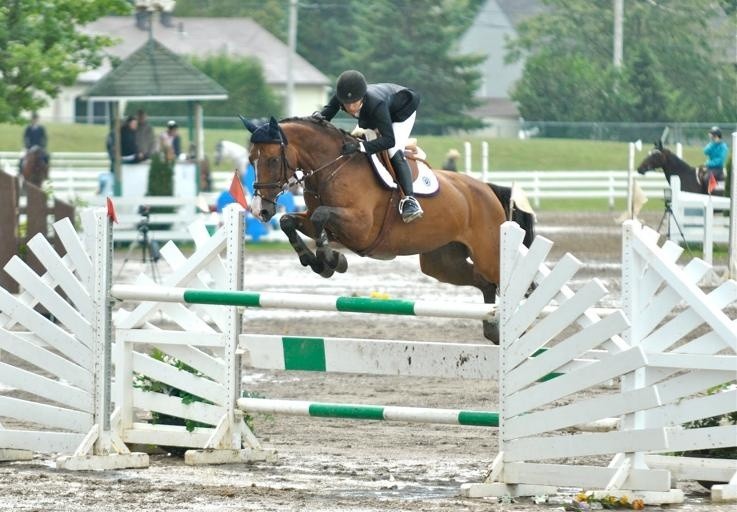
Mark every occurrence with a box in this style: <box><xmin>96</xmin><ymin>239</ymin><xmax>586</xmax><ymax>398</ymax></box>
<box><xmin>107</xmin><ymin>109</ymin><xmax>182</xmax><ymax>172</ymax></box>
<box><xmin>702</xmin><ymin>126</ymin><xmax>729</xmax><ymax>194</ymax></box>
<box><xmin>320</xmin><ymin>70</ymin><xmax>423</xmax><ymax>224</ymax></box>
<box><xmin>20</xmin><ymin>114</ymin><xmax>49</xmax><ymax>180</ymax></box>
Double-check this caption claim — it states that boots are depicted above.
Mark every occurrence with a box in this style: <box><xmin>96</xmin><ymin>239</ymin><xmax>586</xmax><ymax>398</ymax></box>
<box><xmin>389</xmin><ymin>149</ymin><xmax>423</xmax><ymax>224</ymax></box>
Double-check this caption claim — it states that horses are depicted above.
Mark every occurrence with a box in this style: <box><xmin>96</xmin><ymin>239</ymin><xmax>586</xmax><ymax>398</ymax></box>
<box><xmin>637</xmin><ymin>139</ymin><xmax>732</xmax><ymax>215</ymax></box>
<box><xmin>212</xmin><ymin>138</ymin><xmax>303</xmax><ymax>196</ymax></box>
<box><xmin>237</xmin><ymin>112</ymin><xmax>535</xmax><ymax>345</ymax></box>
<box><xmin>20</xmin><ymin>148</ymin><xmax>51</xmax><ymax>189</ymax></box>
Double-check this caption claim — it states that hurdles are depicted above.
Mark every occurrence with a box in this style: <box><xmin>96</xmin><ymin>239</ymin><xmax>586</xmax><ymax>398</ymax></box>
<box><xmin>0</xmin><ymin>207</ymin><xmax>737</xmax><ymax>503</ymax></box>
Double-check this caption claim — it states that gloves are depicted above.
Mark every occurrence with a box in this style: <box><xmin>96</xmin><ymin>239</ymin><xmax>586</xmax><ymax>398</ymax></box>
<box><xmin>342</xmin><ymin>142</ymin><xmax>362</xmax><ymax>155</ymax></box>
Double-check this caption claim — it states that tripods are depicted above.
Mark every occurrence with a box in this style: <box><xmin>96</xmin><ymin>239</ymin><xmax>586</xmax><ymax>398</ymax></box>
<box><xmin>113</xmin><ymin>220</ymin><xmax>163</xmax><ymax>285</ymax></box>
<box><xmin>657</xmin><ymin>206</ymin><xmax>690</xmax><ymax>251</ymax></box>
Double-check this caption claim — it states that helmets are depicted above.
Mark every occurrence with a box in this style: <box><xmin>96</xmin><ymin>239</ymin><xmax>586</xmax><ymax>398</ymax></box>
<box><xmin>335</xmin><ymin>70</ymin><xmax>368</xmax><ymax>102</ymax></box>
<box><xmin>708</xmin><ymin>126</ymin><xmax>721</xmax><ymax>138</ymax></box>
